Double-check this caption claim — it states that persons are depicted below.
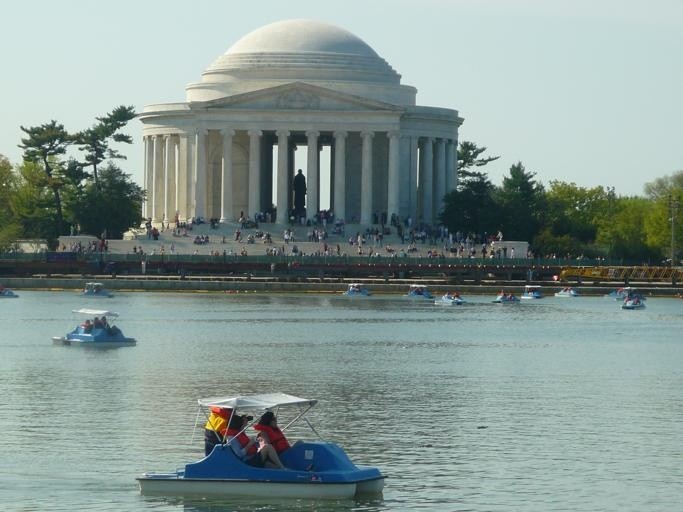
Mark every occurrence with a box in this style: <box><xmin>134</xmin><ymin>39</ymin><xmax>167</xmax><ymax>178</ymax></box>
<box><xmin>204</xmin><ymin>409</ymin><xmax>248</xmax><ymax>458</ymax></box>
<box><xmin>220</xmin><ymin>414</ymin><xmax>287</xmax><ymax>470</ymax></box>
<box><xmin>291</xmin><ymin>167</ymin><xmax>308</xmax><ymax>210</ymax></box>
<box><xmin>0</xmin><ymin>206</ymin><xmax>682</xmax><ymax>305</ymax></box>
<box><xmin>80</xmin><ymin>314</ymin><xmax>111</xmax><ymax>334</ymax></box>
<box><xmin>251</xmin><ymin>410</ymin><xmax>304</xmax><ymax>457</ymax></box>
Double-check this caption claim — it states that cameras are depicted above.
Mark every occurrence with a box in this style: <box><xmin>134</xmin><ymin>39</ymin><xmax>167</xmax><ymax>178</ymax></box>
<box><xmin>247</xmin><ymin>415</ymin><xmax>254</xmax><ymax>421</ymax></box>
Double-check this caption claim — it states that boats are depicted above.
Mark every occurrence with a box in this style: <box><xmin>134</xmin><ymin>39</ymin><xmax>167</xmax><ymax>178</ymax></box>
<box><xmin>81</xmin><ymin>288</ymin><xmax>115</xmax><ymax>298</ymax></box>
<box><xmin>136</xmin><ymin>436</ymin><xmax>389</xmax><ymax>501</ymax></box>
<box><xmin>0</xmin><ymin>290</ymin><xmax>19</xmax><ymax>298</ymax></box>
<box><xmin>52</xmin><ymin>324</ymin><xmax>137</xmax><ymax>349</ymax></box>
